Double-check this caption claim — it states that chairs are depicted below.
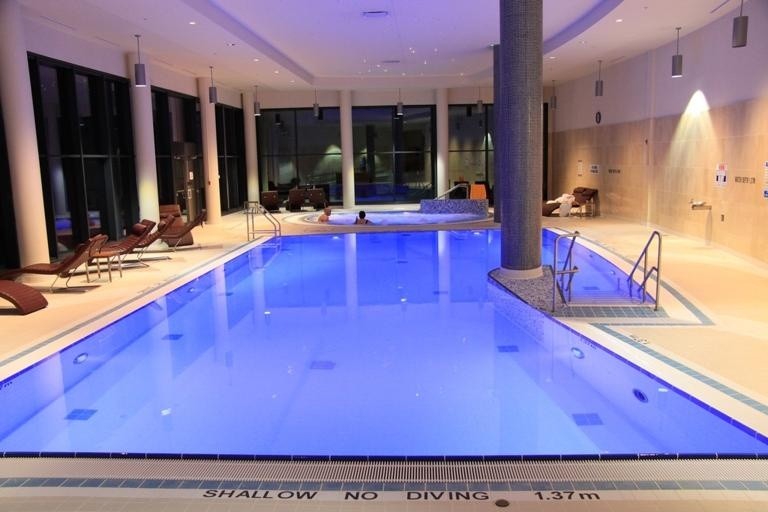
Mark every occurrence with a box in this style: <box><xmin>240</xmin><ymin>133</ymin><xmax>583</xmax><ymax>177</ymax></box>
<box><xmin>261</xmin><ymin>185</ymin><xmax>329</xmax><ymax>214</ymax></box>
<box><xmin>543</xmin><ymin>185</ymin><xmax>598</xmax><ymax>218</ymax></box>
<box><xmin>0</xmin><ymin>203</ymin><xmax>209</xmax><ymax>316</ymax></box>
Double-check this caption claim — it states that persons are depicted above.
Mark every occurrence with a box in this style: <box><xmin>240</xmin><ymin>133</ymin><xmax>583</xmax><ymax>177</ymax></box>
<box><xmin>355</xmin><ymin>211</ymin><xmax>374</xmax><ymax>225</ymax></box>
<box><xmin>318</xmin><ymin>208</ymin><xmax>331</xmax><ymax>223</ymax></box>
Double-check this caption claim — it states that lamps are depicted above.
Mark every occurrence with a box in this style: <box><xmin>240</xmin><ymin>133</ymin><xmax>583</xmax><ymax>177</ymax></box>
<box><xmin>671</xmin><ymin>26</ymin><xmax>683</xmax><ymax>78</ymax></box>
<box><xmin>732</xmin><ymin>0</ymin><xmax>749</xmax><ymax>49</ymax></box>
<box><xmin>313</xmin><ymin>87</ymin><xmax>319</xmax><ymax>117</ymax></box>
<box><xmin>208</xmin><ymin>66</ymin><xmax>218</xmax><ymax>104</ymax></box>
<box><xmin>395</xmin><ymin>88</ymin><xmax>403</xmax><ymax>115</ymax></box>
<box><xmin>134</xmin><ymin>34</ymin><xmax>146</xmax><ymax>88</ymax></box>
<box><xmin>596</xmin><ymin>60</ymin><xmax>604</xmax><ymax>97</ymax></box>
<box><xmin>253</xmin><ymin>85</ymin><xmax>261</xmax><ymax>116</ymax></box>
<box><xmin>549</xmin><ymin>79</ymin><xmax>558</xmax><ymax>108</ymax></box>
